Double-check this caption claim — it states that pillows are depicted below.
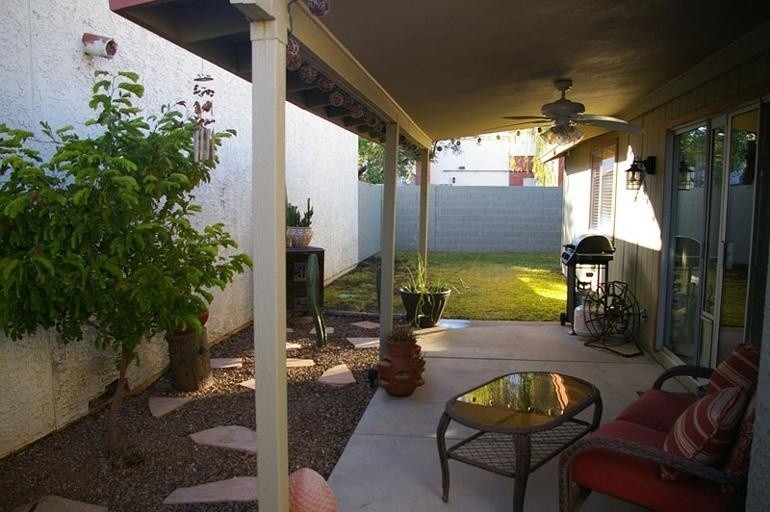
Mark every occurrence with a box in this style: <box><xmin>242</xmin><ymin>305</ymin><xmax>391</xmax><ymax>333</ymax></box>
<box><xmin>658</xmin><ymin>342</ymin><xmax>760</xmax><ymax>502</ymax></box>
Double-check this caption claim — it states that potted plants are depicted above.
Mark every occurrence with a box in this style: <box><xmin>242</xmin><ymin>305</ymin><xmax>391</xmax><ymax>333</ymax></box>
<box><xmin>376</xmin><ymin>328</ymin><xmax>428</xmax><ymax>397</ymax></box>
<box><xmin>399</xmin><ymin>234</ymin><xmax>469</xmax><ymax>330</ymax></box>
<box><xmin>286</xmin><ymin>197</ymin><xmax>314</xmax><ymax>248</ymax></box>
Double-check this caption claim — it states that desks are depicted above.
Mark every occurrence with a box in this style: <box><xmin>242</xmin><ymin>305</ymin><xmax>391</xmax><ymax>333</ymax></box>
<box><xmin>285</xmin><ymin>245</ymin><xmax>325</xmax><ymax>316</ymax></box>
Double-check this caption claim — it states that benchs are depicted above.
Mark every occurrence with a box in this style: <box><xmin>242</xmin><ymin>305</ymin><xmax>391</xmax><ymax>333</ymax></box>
<box><xmin>557</xmin><ymin>363</ymin><xmax>759</xmax><ymax>510</ymax></box>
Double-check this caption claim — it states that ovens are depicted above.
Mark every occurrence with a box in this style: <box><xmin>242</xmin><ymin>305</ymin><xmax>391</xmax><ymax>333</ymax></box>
<box><xmin>560</xmin><ymin>234</ymin><xmax>615</xmax><ymax>266</ymax></box>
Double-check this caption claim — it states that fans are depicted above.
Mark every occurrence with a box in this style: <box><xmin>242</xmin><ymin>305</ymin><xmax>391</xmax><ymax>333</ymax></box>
<box><xmin>481</xmin><ymin>79</ymin><xmax>643</xmax><ymax>145</ymax></box>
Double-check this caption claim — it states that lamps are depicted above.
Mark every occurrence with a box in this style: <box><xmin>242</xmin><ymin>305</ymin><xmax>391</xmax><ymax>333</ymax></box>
<box><xmin>678</xmin><ymin>161</ymin><xmax>697</xmax><ymax>191</ymax></box>
<box><xmin>625</xmin><ymin>156</ymin><xmax>655</xmax><ymax>190</ymax></box>
<box><xmin>540</xmin><ymin>121</ymin><xmax>585</xmax><ymax>145</ymax></box>
